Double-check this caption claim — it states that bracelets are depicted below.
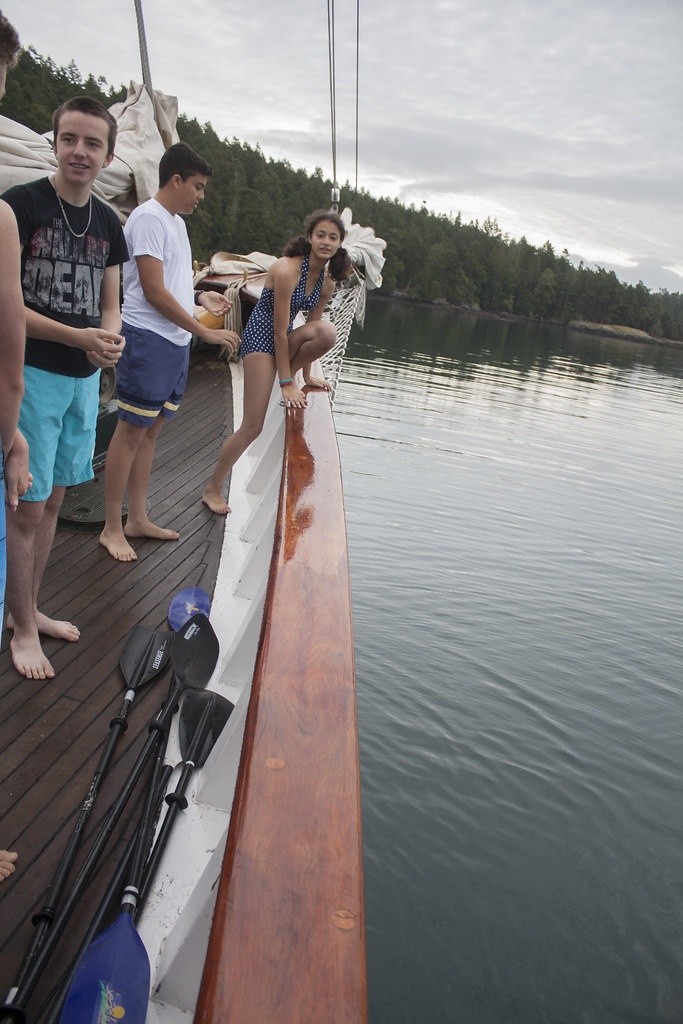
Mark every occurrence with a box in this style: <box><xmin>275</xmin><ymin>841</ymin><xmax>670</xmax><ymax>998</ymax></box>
<box><xmin>278</xmin><ymin>378</ymin><xmax>293</xmax><ymax>384</ymax></box>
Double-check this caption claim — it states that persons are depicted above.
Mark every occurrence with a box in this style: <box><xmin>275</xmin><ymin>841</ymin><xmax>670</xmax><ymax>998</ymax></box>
<box><xmin>1</xmin><ymin>199</ymin><xmax>33</xmax><ymax>634</ymax></box>
<box><xmin>98</xmin><ymin>141</ymin><xmax>242</xmax><ymax>562</ymax></box>
<box><xmin>0</xmin><ymin>96</ymin><xmax>131</xmax><ymax>681</ymax></box>
<box><xmin>201</xmin><ymin>208</ymin><xmax>353</xmax><ymax>514</ymax></box>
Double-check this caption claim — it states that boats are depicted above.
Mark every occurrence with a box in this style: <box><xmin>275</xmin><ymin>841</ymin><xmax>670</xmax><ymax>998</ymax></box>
<box><xmin>0</xmin><ymin>0</ymin><xmax>382</xmax><ymax>1023</ymax></box>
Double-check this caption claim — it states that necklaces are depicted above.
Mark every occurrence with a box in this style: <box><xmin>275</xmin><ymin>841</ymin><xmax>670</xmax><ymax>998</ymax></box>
<box><xmin>52</xmin><ymin>171</ymin><xmax>92</xmax><ymax>238</ymax></box>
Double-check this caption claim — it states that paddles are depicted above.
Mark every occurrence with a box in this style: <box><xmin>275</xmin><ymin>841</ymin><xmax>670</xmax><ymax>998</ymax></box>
<box><xmin>3</xmin><ymin>584</ymin><xmax>235</xmax><ymax>1024</ymax></box>
